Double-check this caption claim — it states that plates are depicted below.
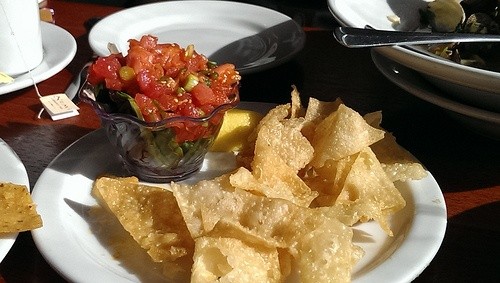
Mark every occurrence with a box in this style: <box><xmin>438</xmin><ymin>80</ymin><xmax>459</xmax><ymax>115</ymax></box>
<box><xmin>0</xmin><ymin>20</ymin><xmax>78</xmax><ymax>95</ymax></box>
<box><xmin>326</xmin><ymin>0</ymin><xmax>500</xmax><ymax>124</ymax></box>
<box><xmin>87</xmin><ymin>0</ymin><xmax>308</xmax><ymax>74</ymax></box>
<box><xmin>0</xmin><ymin>135</ymin><xmax>30</xmax><ymax>263</ymax></box>
<box><xmin>28</xmin><ymin>101</ymin><xmax>449</xmax><ymax>283</ymax></box>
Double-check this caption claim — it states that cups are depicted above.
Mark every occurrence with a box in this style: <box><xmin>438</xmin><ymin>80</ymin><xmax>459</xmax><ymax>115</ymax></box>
<box><xmin>0</xmin><ymin>0</ymin><xmax>44</xmax><ymax>77</ymax></box>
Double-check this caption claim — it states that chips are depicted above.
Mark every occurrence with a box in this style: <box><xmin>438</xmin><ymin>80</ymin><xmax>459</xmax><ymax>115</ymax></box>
<box><xmin>94</xmin><ymin>84</ymin><xmax>430</xmax><ymax>283</ymax></box>
<box><xmin>0</xmin><ymin>182</ymin><xmax>42</xmax><ymax>233</ymax></box>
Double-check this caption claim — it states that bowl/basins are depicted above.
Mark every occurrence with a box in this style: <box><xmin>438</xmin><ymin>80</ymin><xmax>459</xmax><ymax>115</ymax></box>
<box><xmin>76</xmin><ymin>87</ymin><xmax>239</xmax><ymax>183</ymax></box>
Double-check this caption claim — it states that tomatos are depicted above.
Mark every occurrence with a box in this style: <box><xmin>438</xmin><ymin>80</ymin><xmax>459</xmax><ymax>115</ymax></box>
<box><xmin>85</xmin><ymin>34</ymin><xmax>241</xmax><ymax>141</ymax></box>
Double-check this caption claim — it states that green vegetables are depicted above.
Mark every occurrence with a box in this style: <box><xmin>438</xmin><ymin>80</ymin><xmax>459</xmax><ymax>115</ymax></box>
<box><xmin>416</xmin><ymin>0</ymin><xmax>500</xmax><ymax>72</ymax></box>
<box><xmin>95</xmin><ymin>87</ymin><xmax>218</xmax><ymax>171</ymax></box>
